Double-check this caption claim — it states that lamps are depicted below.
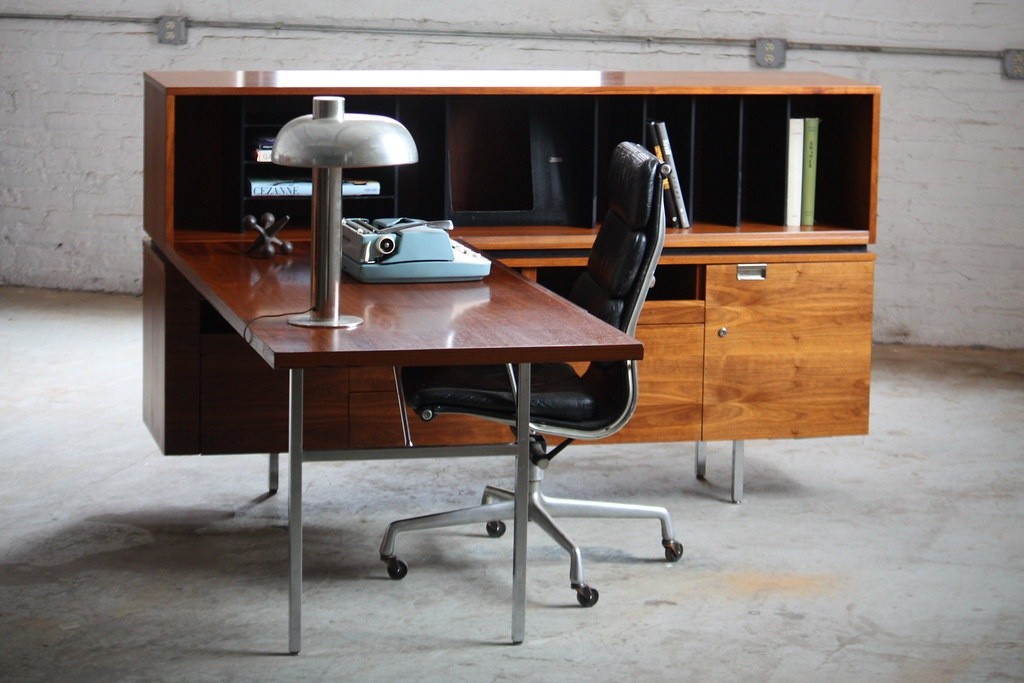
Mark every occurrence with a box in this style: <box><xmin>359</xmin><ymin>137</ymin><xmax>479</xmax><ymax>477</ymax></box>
<box><xmin>269</xmin><ymin>96</ymin><xmax>419</xmax><ymax>327</ymax></box>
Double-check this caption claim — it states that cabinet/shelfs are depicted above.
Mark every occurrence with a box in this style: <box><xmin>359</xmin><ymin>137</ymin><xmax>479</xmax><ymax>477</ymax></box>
<box><xmin>141</xmin><ymin>71</ymin><xmax>882</xmax><ymax>504</ymax></box>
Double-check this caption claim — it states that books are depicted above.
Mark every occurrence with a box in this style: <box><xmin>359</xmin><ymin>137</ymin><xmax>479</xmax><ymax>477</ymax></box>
<box><xmin>787</xmin><ymin>117</ymin><xmax>818</xmax><ymax>226</ymax></box>
<box><xmin>646</xmin><ymin>119</ymin><xmax>689</xmax><ymax>228</ymax></box>
<box><xmin>255</xmin><ymin>137</ymin><xmax>276</xmax><ymax>163</ymax></box>
<box><xmin>248</xmin><ymin>177</ymin><xmax>380</xmax><ymax>196</ymax></box>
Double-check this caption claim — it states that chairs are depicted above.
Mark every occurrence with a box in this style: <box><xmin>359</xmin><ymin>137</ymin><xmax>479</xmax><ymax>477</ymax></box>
<box><xmin>379</xmin><ymin>142</ymin><xmax>683</xmax><ymax>609</ymax></box>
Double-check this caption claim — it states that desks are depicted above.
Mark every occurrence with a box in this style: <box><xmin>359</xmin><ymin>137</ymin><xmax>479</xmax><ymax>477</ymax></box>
<box><xmin>164</xmin><ymin>240</ymin><xmax>644</xmax><ymax>655</ymax></box>
